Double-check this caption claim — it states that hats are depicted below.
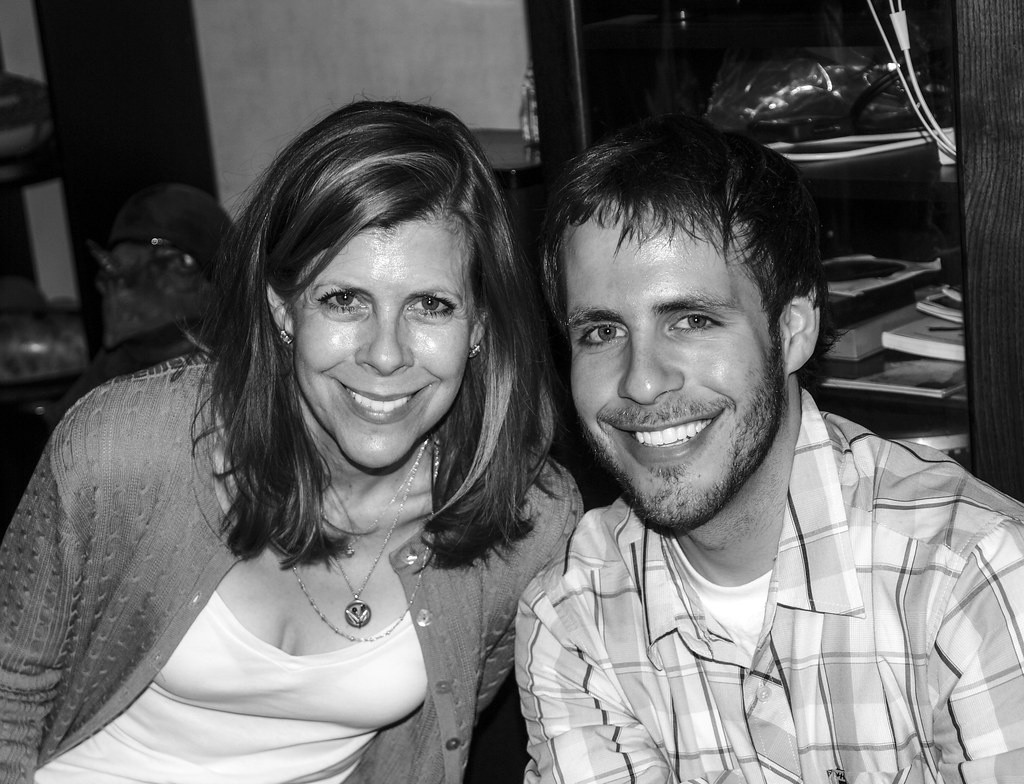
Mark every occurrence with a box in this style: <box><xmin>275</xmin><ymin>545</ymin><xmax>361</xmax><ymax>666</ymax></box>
<box><xmin>107</xmin><ymin>180</ymin><xmax>236</xmax><ymax>278</ymax></box>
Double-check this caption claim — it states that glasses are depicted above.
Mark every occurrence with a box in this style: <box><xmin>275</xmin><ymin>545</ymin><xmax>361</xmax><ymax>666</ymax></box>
<box><xmin>96</xmin><ymin>249</ymin><xmax>203</xmax><ymax>301</ymax></box>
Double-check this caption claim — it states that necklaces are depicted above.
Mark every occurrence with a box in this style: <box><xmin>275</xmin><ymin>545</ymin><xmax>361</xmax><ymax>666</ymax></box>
<box><xmin>293</xmin><ymin>433</ymin><xmax>443</xmax><ymax>644</ymax></box>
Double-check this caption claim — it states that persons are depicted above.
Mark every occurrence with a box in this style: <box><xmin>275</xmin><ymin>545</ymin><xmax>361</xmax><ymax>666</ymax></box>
<box><xmin>1</xmin><ymin>99</ymin><xmax>584</xmax><ymax>784</ymax></box>
<box><xmin>512</xmin><ymin>120</ymin><xmax>1024</xmax><ymax>783</ymax></box>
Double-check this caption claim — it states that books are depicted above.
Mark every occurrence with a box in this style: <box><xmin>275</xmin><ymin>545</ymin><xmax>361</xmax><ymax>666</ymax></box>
<box><xmin>808</xmin><ymin>256</ymin><xmax>967</xmax><ymax>398</ymax></box>
